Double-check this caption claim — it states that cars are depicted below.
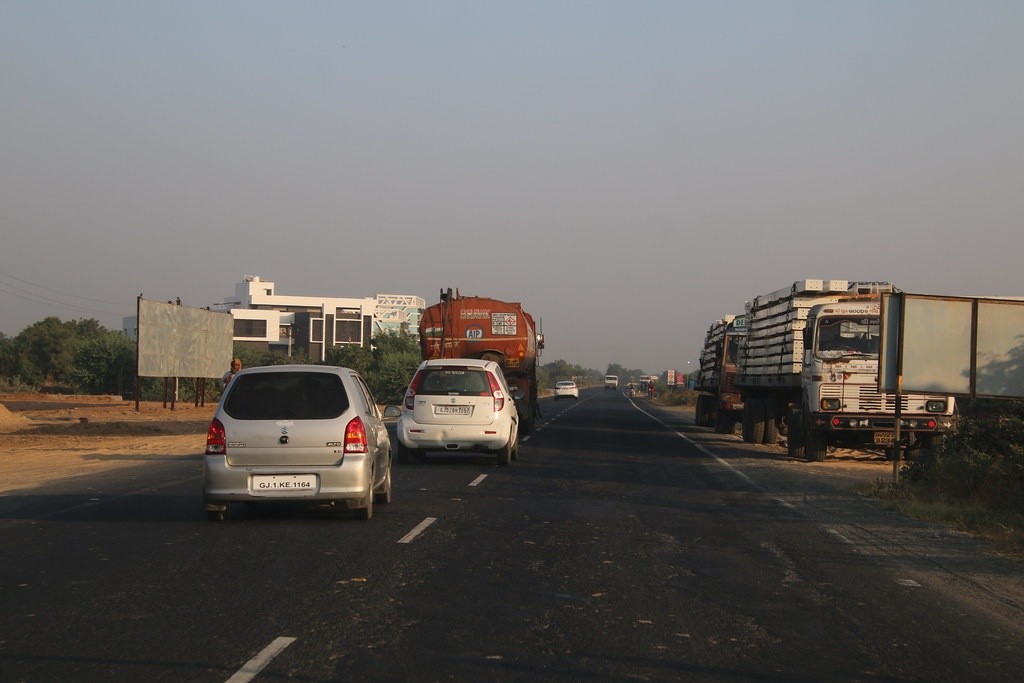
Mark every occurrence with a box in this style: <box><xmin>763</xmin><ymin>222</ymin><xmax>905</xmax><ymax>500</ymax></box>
<box><xmin>396</xmin><ymin>357</ymin><xmax>519</xmax><ymax>467</ymax></box>
<box><xmin>554</xmin><ymin>381</ymin><xmax>579</xmax><ymax>401</ymax></box>
<box><xmin>202</xmin><ymin>364</ymin><xmax>400</xmax><ymax>521</ymax></box>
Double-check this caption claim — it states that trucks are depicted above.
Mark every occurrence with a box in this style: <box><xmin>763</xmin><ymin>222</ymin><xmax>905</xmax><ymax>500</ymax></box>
<box><xmin>604</xmin><ymin>375</ymin><xmax>620</xmax><ymax>389</ymax></box>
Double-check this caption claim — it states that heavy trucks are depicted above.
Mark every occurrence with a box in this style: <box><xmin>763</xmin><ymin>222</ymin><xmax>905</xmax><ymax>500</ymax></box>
<box><xmin>686</xmin><ymin>314</ymin><xmax>751</xmax><ymax>437</ymax></box>
<box><xmin>735</xmin><ymin>278</ymin><xmax>954</xmax><ymax>465</ymax></box>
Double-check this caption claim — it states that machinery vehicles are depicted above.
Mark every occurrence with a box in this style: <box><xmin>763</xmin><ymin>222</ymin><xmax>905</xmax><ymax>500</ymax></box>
<box><xmin>415</xmin><ymin>288</ymin><xmax>545</xmax><ymax>436</ymax></box>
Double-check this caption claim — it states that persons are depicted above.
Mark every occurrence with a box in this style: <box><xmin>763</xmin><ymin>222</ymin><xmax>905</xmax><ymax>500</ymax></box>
<box><xmin>648</xmin><ymin>381</ymin><xmax>655</xmax><ymax>399</ymax></box>
<box><xmin>219</xmin><ymin>356</ymin><xmax>242</xmax><ymax>392</ymax></box>
